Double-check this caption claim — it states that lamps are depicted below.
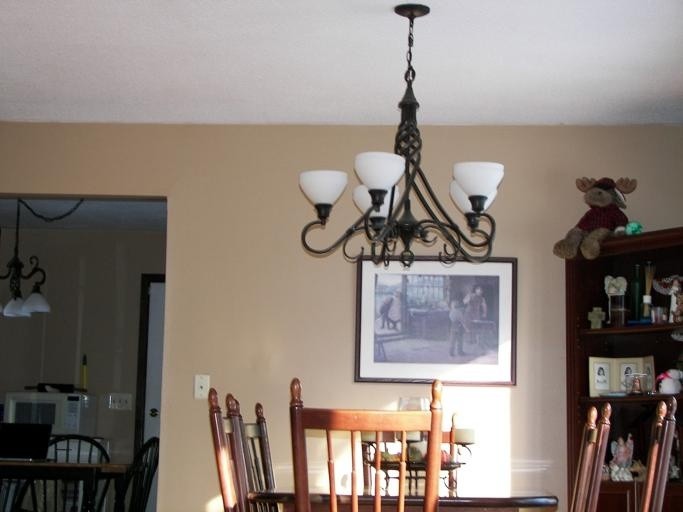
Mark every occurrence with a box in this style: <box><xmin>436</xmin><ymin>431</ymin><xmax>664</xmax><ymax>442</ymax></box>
<box><xmin>299</xmin><ymin>5</ymin><xmax>505</xmax><ymax>270</ymax></box>
<box><xmin>1</xmin><ymin>194</ymin><xmax>86</xmax><ymax>320</ymax></box>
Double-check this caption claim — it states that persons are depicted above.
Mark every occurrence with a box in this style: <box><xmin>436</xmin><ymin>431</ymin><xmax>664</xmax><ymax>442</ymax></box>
<box><xmin>596</xmin><ymin>366</ymin><xmax>608</xmax><ymax>386</ymax></box>
<box><xmin>651</xmin><ymin>274</ymin><xmax>683</xmax><ymax>323</ymax></box>
<box><xmin>463</xmin><ymin>287</ymin><xmax>488</xmax><ymax>344</ymax></box>
<box><xmin>608</xmin><ymin>431</ymin><xmax>635</xmax><ymax>482</ymax></box>
<box><xmin>380</xmin><ymin>289</ymin><xmax>402</xmax><ymax>330</ymax></box>
<box><xmin>621</xmin><ymin>366</ymin><xmax>634</xmax><ymax>389</ymax></box>
<box><xmin>448</xmin><ymin>300</ymin><xmax>470</xmax><ymax>357</ymax></box>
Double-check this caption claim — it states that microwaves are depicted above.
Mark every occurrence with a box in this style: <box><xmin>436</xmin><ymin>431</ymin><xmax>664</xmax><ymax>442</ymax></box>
<box><xmin>4</xmin><ymin>391</ymin><xmax>81</xmax><ymax>452</ymax></box>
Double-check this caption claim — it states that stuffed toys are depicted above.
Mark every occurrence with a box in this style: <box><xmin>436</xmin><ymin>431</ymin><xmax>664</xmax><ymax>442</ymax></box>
<box><xmin>658</xmin><ymin>369</ymin><xmax>683</xmax><ymax>395</ymax></box>
<box><xmin>552</xmin><ymin>176</ymin><xmax>639</xmax><ymax>260</ymax></box>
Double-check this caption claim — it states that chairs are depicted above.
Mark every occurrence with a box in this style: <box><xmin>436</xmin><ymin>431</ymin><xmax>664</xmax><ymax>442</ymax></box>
<box><xmin>355</xmin><ymin>429</ymin><xmax>458</xmax><ymax>498</ymax></box>
<box><xmin>567</xmin><ymin>400</ymin><xmax>614</xmax><ymax>512</ymax></box>
<box><xmin>30</xmin><ymin>432</ymin><xmax>110</xmax><ymax>511</ymax></box>
<box><xmin>207</xmin><ymin>388</ymin><xmax>279</xmax><ymax>511</ymax></box>
<box><xmin>237</xmin><ymin>400</ymin><xmax>276</xmax><ymax>511</ymax></box>
<box><xmin>639</xmin><ymin>394</ymin><xmax>677</xmax><ymax>512</ymax></box>
<box><xmin>116</xmin><ymin>433</ymin><xmax>160</xmax><ymax>511</ymax></box>
<box><xmin>287</xmin><ymin>374</ymin><xmax>447</xmax><ymax>512</ymax></box>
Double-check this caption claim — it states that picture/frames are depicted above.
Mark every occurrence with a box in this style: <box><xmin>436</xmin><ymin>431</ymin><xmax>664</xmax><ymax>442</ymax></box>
<box><xmin>353</xmin><ymin>256</ymin><xmax>518</xmax><ymax>387</ymax></box>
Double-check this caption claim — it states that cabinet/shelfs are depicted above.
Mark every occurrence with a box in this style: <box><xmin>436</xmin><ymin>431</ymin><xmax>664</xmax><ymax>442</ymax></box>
<box><xmin>565</xmin><ymin>225</ymin><xmax>683</xmax><ymax>512</ymax></box>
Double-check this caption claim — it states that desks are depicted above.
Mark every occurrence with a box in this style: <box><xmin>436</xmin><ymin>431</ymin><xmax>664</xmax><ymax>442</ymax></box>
<box><xmin>1</xmin><ymin>459</ymin><xmax>126</xmax><ymax>512</ymax></box>
<box><xmin>244</xmin><ymin>489</ymin><xmax>558</xmax><ymax>511</ymax></box>
